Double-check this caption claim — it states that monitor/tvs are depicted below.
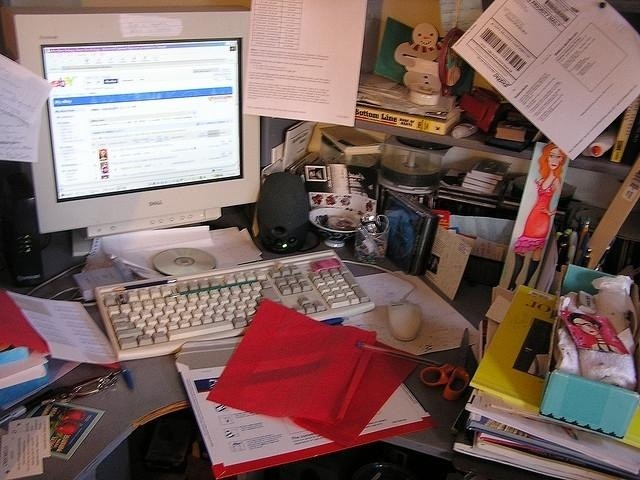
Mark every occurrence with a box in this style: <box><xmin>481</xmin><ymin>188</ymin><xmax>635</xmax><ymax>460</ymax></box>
<box><xmin>8</xmin><ymin>4</ymin><xmax>261</xmax><ymax>258</ymax></box>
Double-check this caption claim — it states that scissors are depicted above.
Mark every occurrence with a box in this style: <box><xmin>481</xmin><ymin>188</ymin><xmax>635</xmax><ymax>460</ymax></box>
<box><xmin>420</xmin><ymin>328</ymin><xmax>471</xmax><ymax>401</ymax></box>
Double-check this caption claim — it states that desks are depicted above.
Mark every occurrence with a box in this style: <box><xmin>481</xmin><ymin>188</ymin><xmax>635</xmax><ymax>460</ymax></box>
<box><xmin>1</xmin><ymin>1</ymin><xmax>640</xmax><ymax>479</ymax></box>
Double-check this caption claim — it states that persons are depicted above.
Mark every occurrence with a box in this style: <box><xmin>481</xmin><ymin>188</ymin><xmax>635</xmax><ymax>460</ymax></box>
<box><xmin>507</xmin><ymin>143</ymin><xmax>569</xmax><ymax>291</ymax></box>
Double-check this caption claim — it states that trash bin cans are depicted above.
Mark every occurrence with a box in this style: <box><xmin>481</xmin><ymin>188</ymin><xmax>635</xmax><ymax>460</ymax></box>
<box><xmin>353</xmin><ymin>449</ymin><xmax>413</xmax><ymax>479</ymax></box>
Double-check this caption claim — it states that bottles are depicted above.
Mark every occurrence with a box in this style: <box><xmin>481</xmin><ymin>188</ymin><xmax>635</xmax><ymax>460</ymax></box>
<box><xmin>582</xmin><ymin>129</ymin><xmax>616</xmax><ymax>157</ymax></box>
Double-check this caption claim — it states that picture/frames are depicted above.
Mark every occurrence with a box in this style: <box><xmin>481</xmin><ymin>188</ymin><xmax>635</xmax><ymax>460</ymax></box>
<box><xmin>380</xmin><ymin>189</ymin><xmax>440</xmax><ymax>276</ymax></box>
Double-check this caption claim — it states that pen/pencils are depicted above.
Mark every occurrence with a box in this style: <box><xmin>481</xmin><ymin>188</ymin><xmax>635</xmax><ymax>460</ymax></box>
<box><xmin>0</xmin><ymin>389</ymin><xmax>53</xmax><ymax>428</ymax></box>
<box><xmin>169</xmin><ymin>280</ymin><xmax>259</xmax><ymax>298</ymax></box>
<box><xmin>556</xmin><ymin>217</ymin><xmax>616</xmax><ymax>272</ymax></box>
<box><xmin>356</xmin><ymin>341</ymin><xmax>441</xmax><ymax>366</ymax></box>
<box><xmin>115</xmin><ymin>279</ymin><xmax>176</xmax><ymax>291</ymax></box>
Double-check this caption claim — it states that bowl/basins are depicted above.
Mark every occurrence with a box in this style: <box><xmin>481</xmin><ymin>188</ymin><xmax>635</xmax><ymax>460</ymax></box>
<box><xmin>309</xmin><ymin>207</ymin><xmax>361</xmax><ymax>248</ymax></box>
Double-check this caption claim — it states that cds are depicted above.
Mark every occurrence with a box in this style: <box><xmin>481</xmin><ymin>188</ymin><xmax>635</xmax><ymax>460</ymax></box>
<box><xmin>153</xmin><ymin>248</ymin><xmax>216</xmax><ymax>276</ymax></box>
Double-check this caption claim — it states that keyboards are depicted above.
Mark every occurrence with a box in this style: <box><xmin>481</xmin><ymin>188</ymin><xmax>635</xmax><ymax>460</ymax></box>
<box><xmin>94</xmin><ymin>248</ymin><xmax>376</xmax><ymax>362</ymax></box>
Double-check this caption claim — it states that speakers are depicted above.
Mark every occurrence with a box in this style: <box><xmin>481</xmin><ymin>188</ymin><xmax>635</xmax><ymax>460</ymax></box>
<box><xmin>4</xmin><ymin>173</ymin><xmax>43</xmax><ymax>287</ymax></box>
<box><xmin>256</xmin><ymin>171</ymin><xmax>309</xmax><ymax>254</ymax></box>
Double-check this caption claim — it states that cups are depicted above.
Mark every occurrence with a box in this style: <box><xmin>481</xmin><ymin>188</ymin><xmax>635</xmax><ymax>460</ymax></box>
<box><xmin>354</xmin><ymin>229</ymin><xmax>389</xmax><ymax>266</ymax></box>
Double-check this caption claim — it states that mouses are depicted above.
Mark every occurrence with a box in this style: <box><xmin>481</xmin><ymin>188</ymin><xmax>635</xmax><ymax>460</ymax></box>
<box><xmin>388</xmin><ymin>299</ymin><xmax>422</xmax><ymax>342</ymax></box>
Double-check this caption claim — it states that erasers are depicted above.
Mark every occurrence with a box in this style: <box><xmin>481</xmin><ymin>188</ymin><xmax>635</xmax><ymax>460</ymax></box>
<box><xmin>311</xmin><ymin>258</ymin><xmax>341</xmax><ymax>272</ymax></box>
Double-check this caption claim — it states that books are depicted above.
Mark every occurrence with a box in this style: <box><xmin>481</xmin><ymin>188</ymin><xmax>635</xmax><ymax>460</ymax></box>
<box><xmin>0</xmin><ymin>288</ymin><xmax>117</xmax><ymax>391</ymax></box>
<box><xmin>252</xmin><ymin>150</ymin><xmax>319</xmax><ymax>238</ymax></box>
<box><xmin>320</xmin><ymin>125</ymin><xmax>382</xmax><ymax>156</ymax></box>
<box><xmin>355</xmin><ymin>72</ymin><xmax>462</xmax><ymax>136</ymax></box>
<box><xmin>609</xmin><ymin>96</ymin><xmax>640</xmax><ymax>163</ymax></box>
<box><xmin>451</xmin><ymin>285</ymin><xmax>640</xmax><ymax>480</ymax></box>
<box><xmin>436</xmin><ymin>158</ymin><xmax>575</xmax><ymax>215</ymax></box>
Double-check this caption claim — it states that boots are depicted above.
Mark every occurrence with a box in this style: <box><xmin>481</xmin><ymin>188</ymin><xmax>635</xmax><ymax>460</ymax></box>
<box><xmin>507</xmin><ymin>254</ymin><xmax>540</xmax><ymax>292</ymax></box>
